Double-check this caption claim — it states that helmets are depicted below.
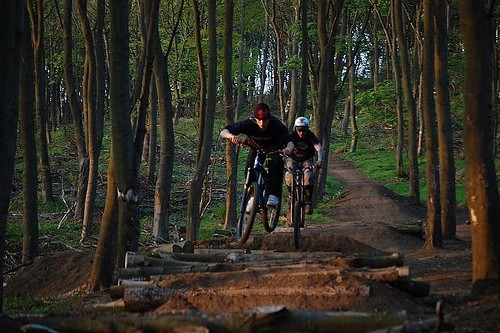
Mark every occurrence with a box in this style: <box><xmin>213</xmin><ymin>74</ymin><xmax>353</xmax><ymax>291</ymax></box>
<box><xmin>294</xmin><ymin>116</ymin><xmax>310</xmax><ymax>127</ymax></box>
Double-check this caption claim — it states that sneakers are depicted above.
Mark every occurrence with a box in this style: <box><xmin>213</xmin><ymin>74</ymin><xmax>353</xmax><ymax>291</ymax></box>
<box><xmin>266</xmin><ymin>195</ymin><xmax>279</xmax><ymax>208</ymax></box>
<box><xmin>246</xmin><ymin>196</ymin><xmax>254</xmax><ymax>213</ymax></box>
<box><xmin>305</xmin><ymin>202</ymin><xmax>313</xmax><ymax>215</ymax></box>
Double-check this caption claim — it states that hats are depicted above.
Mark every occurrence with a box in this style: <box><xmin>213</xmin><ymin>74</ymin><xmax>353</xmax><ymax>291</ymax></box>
<box><xmin>254</xmin><ymin>103</ymin><xmax>271</xmax><ymax>120</ymax></box>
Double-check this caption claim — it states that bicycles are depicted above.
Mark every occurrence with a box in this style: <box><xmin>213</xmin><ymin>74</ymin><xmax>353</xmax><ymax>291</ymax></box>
<box><xmin>235</xmin><ymin>140</ymin><xmax>288</xmax><ymax>243</ymax></box>
<box><xmin>284</xmin><ymin>165</ymin><xmax>315</xmax><ymax>249</ymax></box>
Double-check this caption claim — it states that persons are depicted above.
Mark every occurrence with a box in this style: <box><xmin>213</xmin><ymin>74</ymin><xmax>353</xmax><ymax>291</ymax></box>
<box><xmin>282</xmin><ymin>116</ymin><xmax>323</xmax><ymax>227</ymax></box>
<box><xmin>220</xmin><ymin>103</ymin><xmax>294</xmax><ymax>212</ymax></box>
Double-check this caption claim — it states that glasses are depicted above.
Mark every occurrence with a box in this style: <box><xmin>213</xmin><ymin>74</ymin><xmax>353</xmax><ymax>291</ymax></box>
<box><xmin>296</xmin><ymin>127</ymin><xmax>308</xmax><ymax>132</ymax></box>
